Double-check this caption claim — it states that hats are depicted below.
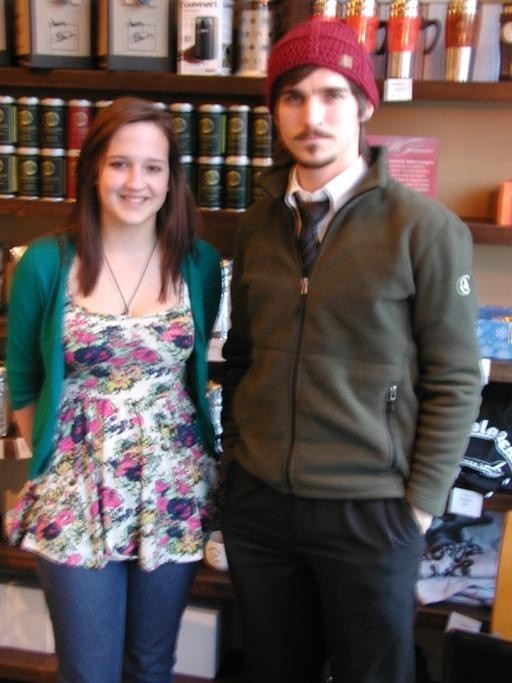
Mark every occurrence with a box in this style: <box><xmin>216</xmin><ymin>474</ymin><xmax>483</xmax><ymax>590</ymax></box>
<box><xmin>267</xmin><ymin>19</ymin><xmax>380</xmax><ymax>108</ymax></box>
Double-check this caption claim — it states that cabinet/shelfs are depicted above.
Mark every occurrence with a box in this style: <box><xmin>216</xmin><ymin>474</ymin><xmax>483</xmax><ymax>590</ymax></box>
<box><xmin>1</xmin><ymin>63</ymin><xmax>512</xmax><ymax>252</ymax></box>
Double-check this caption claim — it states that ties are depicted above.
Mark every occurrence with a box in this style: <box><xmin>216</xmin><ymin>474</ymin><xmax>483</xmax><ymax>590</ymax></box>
<box><xmin>292</xmin><ymin>193</ymin><xmax>329</xmax><ymax>278</ymax></box>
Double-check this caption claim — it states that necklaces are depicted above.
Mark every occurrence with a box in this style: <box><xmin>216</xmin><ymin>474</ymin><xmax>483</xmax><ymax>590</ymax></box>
<box><xmin>94</xmin><ymin>230</ymin><xmax>159</xmax><ymax>317</ymax></box>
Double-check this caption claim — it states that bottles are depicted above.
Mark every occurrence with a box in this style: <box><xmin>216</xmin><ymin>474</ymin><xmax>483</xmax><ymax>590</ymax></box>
<box><xmin>194</xmin><ymin>15</ymin><xmax>215</xmax><ymax>57</ymax></box>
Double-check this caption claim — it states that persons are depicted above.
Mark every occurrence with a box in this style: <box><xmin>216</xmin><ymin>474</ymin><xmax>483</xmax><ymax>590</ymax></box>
<box><xmin>4</xmin><ymin>97</ymin><xmax>221</xmax><ymax>683</ymax></box>
<box><xmin>220</xmin><ymin>15</ymin><xmax>482</xmax><ymax>680</ymax></box>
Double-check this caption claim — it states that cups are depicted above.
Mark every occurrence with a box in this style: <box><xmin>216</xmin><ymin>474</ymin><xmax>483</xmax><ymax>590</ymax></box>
<box><xmin>312</xmin><ymin>0</ymin><xmax>486</xmax><ymax>78</ymax></box>
<box><xmin>236</xmin><ymin>7</ymin><xmax>275</xmax><ymax>78</ymax></box>
